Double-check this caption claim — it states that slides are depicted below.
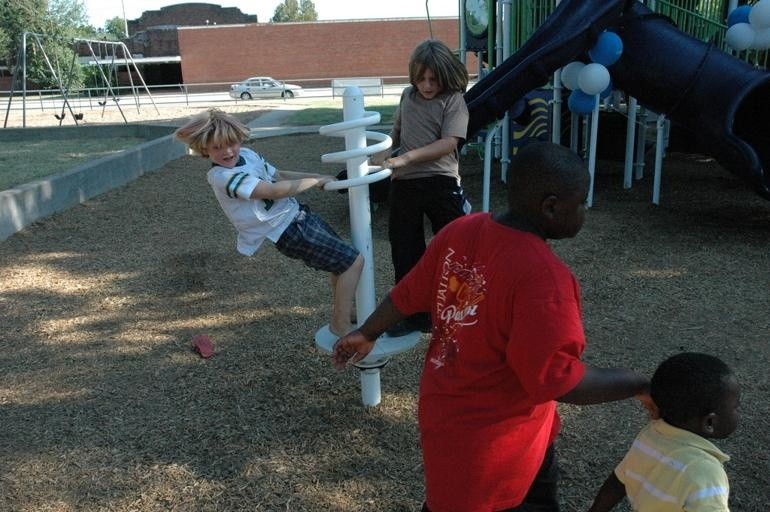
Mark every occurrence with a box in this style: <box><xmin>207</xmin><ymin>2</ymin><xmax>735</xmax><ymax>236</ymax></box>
<box><xmin>334</xmin><ymin>0</ymin><xmax>768</xmax><ymax>198</ymax></box>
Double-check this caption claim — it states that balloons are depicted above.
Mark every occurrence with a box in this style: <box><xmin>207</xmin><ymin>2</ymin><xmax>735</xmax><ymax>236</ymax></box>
<box><xmin>724</xmin><ymin>22</ymin><xmax>756</xmax><ymax>51</ymax></box>
<box><xmin>561</xmin><ymin>61</ymin><xmax>588</xmax><ymax>89</ymax></box>
<box><xmin>749</xmin><ymin>0</ymin><xmax>770</xmax><ymax>30</ymax></box>
<box><xmin>751</xmin><ymin>27</ymin><xmax>770</xmax><ymax>50</ymax></box>
<box><xmin>567</xmin><ymin>90</ymin><xmax>596</xmax><ymax>115</ymax></box>
<box><xmin>727</xmin><ymin>4</ymin><xmax>753</xmax><ymax>28</ymax></box>
<box><xmin>579</xmin><ymin>63</ymin><xmax>611</xmax><ymax>95</ymax></box>
<box><xmin>588</xmin><ymin>31</ymin><xmax>624</xmax><ymax>66</ymax></box>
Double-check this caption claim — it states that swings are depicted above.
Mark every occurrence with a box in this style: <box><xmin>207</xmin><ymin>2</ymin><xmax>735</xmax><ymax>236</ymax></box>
<box><xmin>43</xmin><ymin>38</ymin><xmax>121</xmax><ymax>120</ymax></box>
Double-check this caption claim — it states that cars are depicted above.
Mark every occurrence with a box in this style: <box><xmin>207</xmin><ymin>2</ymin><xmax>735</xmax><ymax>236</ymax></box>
<box><xmin>229</xmin><ymin>76</ymin><xmax>303</xmax><ymax>99</ymax></box>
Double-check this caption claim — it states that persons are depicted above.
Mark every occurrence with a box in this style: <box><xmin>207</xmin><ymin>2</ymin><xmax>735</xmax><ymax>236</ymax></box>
<box><xmin>331</xmin><ymin>142</ymin><xmax>659</xmax><ymax>511</ymax></box>
<box><xmin>369</xmin><ymin>37</ymin><xmax>469</xmax><ymax>337</ymax></box>
<box><xmin>174</xmin><ymin>106</ymin><xmax>365</xmax><ymax>338</ymax></box>
<box><xmin>584</xmin><ymin>351</ymin><xmax>741</xmax><ymax>511</ymax></box>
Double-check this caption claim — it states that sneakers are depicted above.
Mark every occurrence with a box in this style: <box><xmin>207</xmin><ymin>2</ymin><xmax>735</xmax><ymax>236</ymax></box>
<box><xmin>384</xmin><ymin>308</ymin><xmax>430</xmax><ymax>338</ymax></box>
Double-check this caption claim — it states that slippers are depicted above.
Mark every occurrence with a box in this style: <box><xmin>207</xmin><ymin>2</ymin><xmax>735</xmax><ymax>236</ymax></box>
<box><xmin>188</xmin><ymin>334</ymin><xmax>220</xmax><ymax>360</ymax></box>
